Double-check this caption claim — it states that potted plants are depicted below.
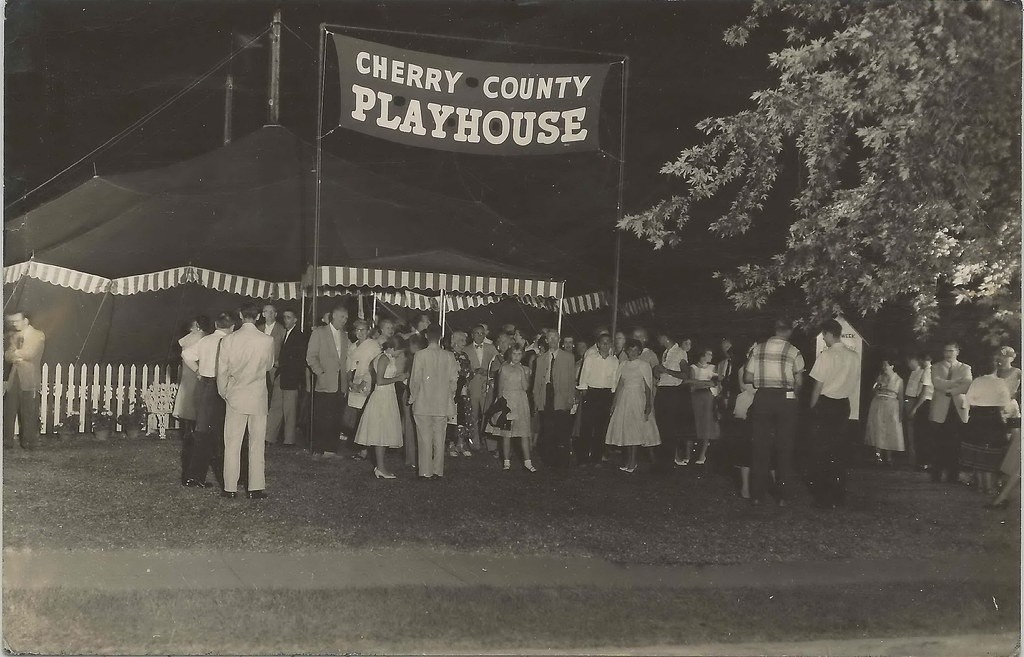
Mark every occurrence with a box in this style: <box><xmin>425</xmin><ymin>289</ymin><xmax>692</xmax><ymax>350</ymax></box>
<box><xmin>117</xmin><ymin>402</ymin><xmax>147</xmax><ymax>437</ymax></box>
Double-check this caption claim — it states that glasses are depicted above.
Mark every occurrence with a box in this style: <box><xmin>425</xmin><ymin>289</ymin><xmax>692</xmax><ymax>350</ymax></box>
<box><xmin>355</xmin><ymin>328</ymin><xmax>367</xmax><ymax>332</ymax></box>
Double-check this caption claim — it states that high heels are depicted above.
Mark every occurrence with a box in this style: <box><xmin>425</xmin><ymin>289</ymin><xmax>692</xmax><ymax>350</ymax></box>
<box><xmin>374</xmin><ymin>467</ymin><xmax>396</xmax><ymax>479</ymax></box>
<box><xmin>983</xmin><ymin>500</ymin><xmax>1009</xmax><ymax>511</ymax></box>
<box><xmin>620</xmin><ymin>464</ymin><xmax>638</xmax><ymax>473</ymax></box>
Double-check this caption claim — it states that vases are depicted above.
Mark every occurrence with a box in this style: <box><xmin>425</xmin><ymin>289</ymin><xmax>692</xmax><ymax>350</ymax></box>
<box><xmin>57</xmin><ymin>432</ymin><xmax>75</xmax><ymax>441</ymax></box>
<box><xmin>93</xmin><ymin>428</ymin><xmax>109</xmax><ymax>440</ymax></box>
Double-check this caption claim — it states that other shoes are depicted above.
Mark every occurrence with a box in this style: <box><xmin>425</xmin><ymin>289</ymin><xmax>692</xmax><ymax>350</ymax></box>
<box><xmin>313</xmin><ymin>452</ymin><xmax>345</xmax><ymax>463</ymax></box>
<box><xmin>524</xmin><ymin>460</ymin><xmax>536</xmax><ymax>473</ymax></box>
<box><xmin>449</xmin><ymin>445</ymin><xmax>458</xmax><ymax>457</ymax></box>
<box><xmin>502</xmin><ymin>460</ymin><xmax>511</xmax><ymax>472</ymax></box>
<box><xmin>673</xmin><ymin>459</ymin><xmax>689</xmax><ymax>466</ymax></box>
<box><xmin>780</xmin><ymin>492</ymin><xmax>800</xmax><ymax>507</ymax></box>
<box><xmin>696</xmin><ymin>457</ymin><xmax>706</xmax><ymax>464</ymax></box>
<box><xmin>456</xmin><ymin>447</ymin><xmax>472</xmax><ymax>458</ymax></box>
<box><xmin>738</xmin><ymin>490</ymin><xmax>754</xmax><ymax>505</ymax></box>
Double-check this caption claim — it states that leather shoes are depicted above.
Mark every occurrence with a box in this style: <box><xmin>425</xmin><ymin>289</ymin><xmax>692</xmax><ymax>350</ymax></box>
<box><xmin>247</xmin><ymin>491</ymin><xmax>267</xmax><ymax>499</ymax></box>
<box><xmin>220</xmin><ymin>491</ymin><xmax>236</xmax><ymax>498</ymax></box>
<box><xmin>186</xmin><ymin>478</ymin><xmax>212</xmax><ymax>488</ymax></box>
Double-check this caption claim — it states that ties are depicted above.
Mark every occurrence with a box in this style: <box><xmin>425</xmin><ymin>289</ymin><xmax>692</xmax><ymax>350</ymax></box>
<box><xmin>550</xmin><ymin>353</ymin><xmax>555</xmax><ymax>385</ymax></box>
<box><xmin>945</xmin><ymin>366</ymin><xmax>952</xmax><ymax>397</ymax></box>
<box><xmin>726</xmin><ymin>360</ymin><xmax>731</xmax><ymax>377</ymax></box>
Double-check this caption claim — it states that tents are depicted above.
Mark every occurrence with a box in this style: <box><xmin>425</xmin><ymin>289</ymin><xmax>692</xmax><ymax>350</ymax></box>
<box><xmin>3</xmin><ymin>124</ymin><xmax>653</xmax><ymax>449</ymax></box>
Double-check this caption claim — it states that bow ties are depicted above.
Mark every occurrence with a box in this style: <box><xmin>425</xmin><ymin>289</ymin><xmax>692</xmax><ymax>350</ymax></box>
<box><xmin>475</xmin><ymin>345</ymin><xmax>483</xmax><ymax>349</ymax></box>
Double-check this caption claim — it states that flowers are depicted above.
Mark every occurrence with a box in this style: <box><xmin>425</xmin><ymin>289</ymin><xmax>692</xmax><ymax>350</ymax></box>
<box><xmin>91</xmin><ymin>408</ymin><xmax>114</xmax><ymax>430</ymax></box>
<box><xmin>53</xmin><ymin>414</ymin><xmax>80</xmax><ymax>432</ymax></box>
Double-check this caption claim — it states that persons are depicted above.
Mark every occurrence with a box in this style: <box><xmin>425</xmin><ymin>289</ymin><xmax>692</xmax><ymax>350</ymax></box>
<box><xmin>865</xmin><ymin>343</ymin><xmax>1022</xmax><ymax>511</ymax></box>
<box><xmin>171</xmin><ymin>301</ymin><xmax>861</xmax><ymax>510</ymax></box>
<box><xmin>3</xmin><ymin>311</ymin><xmax>46</xmax><ymax>450</ymax></box>
